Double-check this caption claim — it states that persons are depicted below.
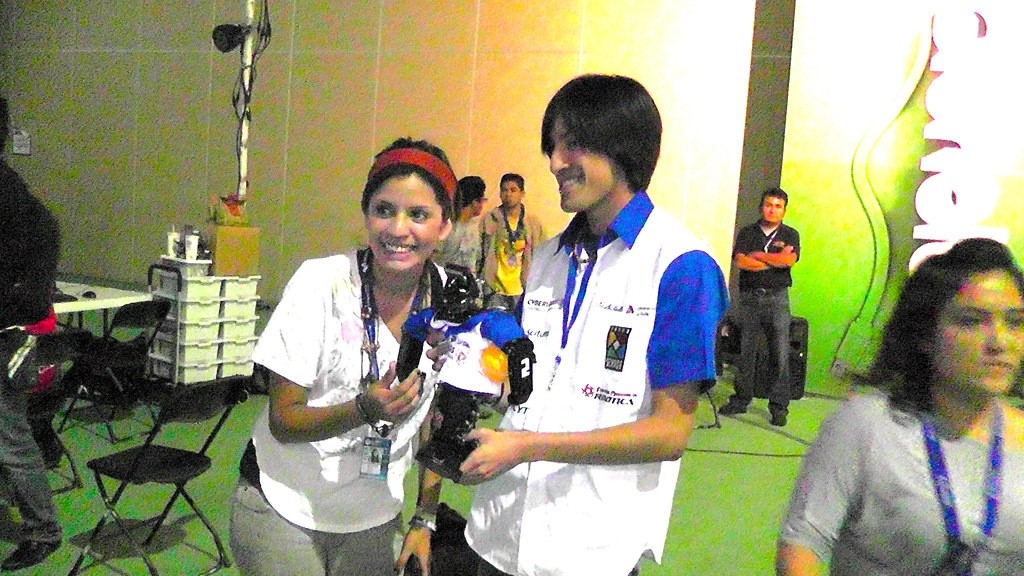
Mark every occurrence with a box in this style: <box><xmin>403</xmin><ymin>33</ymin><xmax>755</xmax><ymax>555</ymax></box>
<box><xmin>229</xmin><ymin>136</ymin><xmax>462</xmax><ymax>575</ymax></box>
<box><xmin>425</xmin><ymin>73</ymin><xmax>730</xmax><ymax>576</ymax></box>
<box><xmin>371</xmin><ymin>449</ymin><xmax>380</xmax><ymax>462</ymax></box>
<box><xmin>774</xmin><ymin>239</ymin><xmax>1024</xmax><ymax>576</ymax></box>
<box><xmin>718</xmin><ymin>187</ymin><xmax>801</xmax><ymax>425</ymax></box>
<box><xmin>397</xmin><ymin>273</ymin><xmax>535</xmax><ymax>478</ymax></box>
<box><xmin>0</xmin><ymin>97</ymin><xmax>62</xmax><ymax>570</ymax></box>
<box><xmin>430</xmin><ymin>176</ymin><xmax>485</xmax><ymax>277</ymax></box>
<box><xmin>478</xmin><ymin>172</ymin><xmax>542</xmax><ymax>305</ymax></box>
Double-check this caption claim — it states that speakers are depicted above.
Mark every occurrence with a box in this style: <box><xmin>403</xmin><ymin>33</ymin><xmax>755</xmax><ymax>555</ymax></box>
<box><xmin>752</xmin><ymin>316</ymin><xmax>808</xmax><ymax>400</ymax></box>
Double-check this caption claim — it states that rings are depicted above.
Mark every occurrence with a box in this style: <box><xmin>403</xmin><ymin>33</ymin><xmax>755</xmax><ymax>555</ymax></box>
<box><xmin>400</xmin><ymin>395</ymin><xmax>410</xmax><ymax>404</ymax></box>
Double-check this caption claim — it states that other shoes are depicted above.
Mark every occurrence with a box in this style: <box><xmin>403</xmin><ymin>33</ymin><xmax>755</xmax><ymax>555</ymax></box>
<box><xmin>767</xmin><ymin>402</ymin><xmax>789</xmax><ymax>427</ymax></box>
<box><xmin>718</xmin><ymin>394</ymin><xmax>748</xmax><ymax>414</ymax></box>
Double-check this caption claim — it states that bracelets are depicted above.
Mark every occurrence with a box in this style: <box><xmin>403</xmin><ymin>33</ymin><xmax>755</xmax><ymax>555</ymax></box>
<box><xmin>355</xmin><ymin>395</ymin><xmax>368</xmax><ymax>419</ymax></box>
<box><xmin>410</xmin><ymin>516</ymin><xmax>438</xmax><ymax>531</ymax></box>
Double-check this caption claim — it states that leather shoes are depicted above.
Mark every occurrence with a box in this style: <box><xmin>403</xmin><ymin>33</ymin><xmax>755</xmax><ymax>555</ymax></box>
<box><xmin>2</xmin><ymin>538</ymin><xmax>62</xmax><ymax>571</ymax></box>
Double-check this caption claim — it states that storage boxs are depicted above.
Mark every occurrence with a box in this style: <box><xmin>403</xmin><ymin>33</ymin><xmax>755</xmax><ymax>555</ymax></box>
<box><xmin>153</xmin><ymin>224</ymin><xmax>261</xmax><ymax>383</ymax></box>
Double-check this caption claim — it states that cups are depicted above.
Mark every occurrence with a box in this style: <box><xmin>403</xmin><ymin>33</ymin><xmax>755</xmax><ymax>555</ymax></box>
<box><xmin>166</xmin><ymin>230</ymin><xmax>179</xmax><ymax>257</ymax></box>
<box><xmin>185</xmin><ymin>235</ymin><xmax>198</xmax><ymax>260</ymax></box>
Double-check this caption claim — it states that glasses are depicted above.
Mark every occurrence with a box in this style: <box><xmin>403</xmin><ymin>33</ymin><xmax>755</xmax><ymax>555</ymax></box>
<box><xmin>476</xmin><ymin>197</ymin><xmax>488</xmax><ymax>203</ymax></box>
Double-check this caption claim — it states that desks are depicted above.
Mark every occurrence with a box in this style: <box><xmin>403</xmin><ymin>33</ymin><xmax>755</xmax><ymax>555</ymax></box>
<box><xmin>52</xmin><ymin>279</ymin><xmax>152</xmax><ymax>337</ymax></box>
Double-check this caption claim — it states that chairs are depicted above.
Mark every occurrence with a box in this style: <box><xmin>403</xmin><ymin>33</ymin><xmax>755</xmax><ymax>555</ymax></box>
<box><xmin>58</xmin><ymin>298</ymin><xmax>171</xmax><ymax>445</ymax></box>
<box><xmin>27</xmin><ymin>358</ymin><xmax>89</xmax><ymax>491</ymax></box>
<box><xmin>66</xmin><ymin>375</ymin><xmax>249</xmax><ymax>576</ymax></box>
